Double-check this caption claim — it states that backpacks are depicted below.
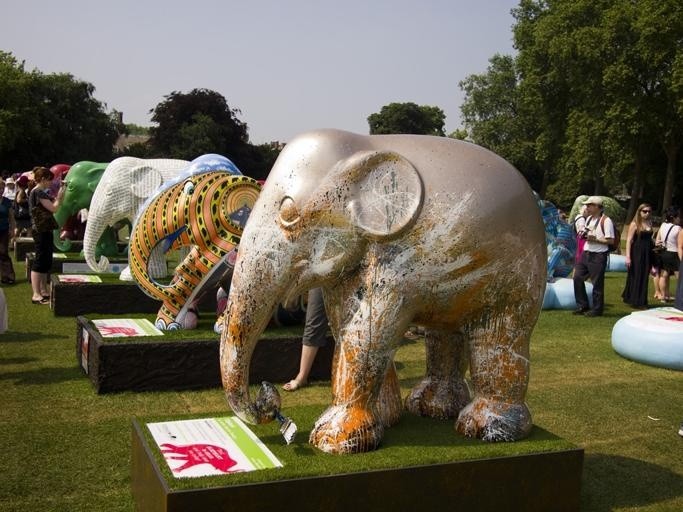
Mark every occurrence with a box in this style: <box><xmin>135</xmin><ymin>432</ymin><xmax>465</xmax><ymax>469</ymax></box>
<box><xmin>570</xmin><ymin>215</ymin><xmax>586</xmax><ymax>235</ymax></box>
<box><xmin>601</xmin><ymin>214</ymin><xmax>621</xmax><ymax>253</ymax></box>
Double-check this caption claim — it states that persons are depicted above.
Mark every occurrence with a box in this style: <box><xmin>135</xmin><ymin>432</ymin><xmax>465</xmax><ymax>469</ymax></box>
<box><xmin>570</xmin><ymin>205</ymin><xmax>588</xmax><ymax>278</ymax></box>
<box><xmin>650</xmin><ymin>215</ymin><xmax>675</xmax><ymax>300</ymax></box>
<box><xmin>623</xmin><ymin>203</ymin><xmax>655</xmax><ymax>310</ymax></box>
<box><xmin>571</xmin><ymin>196</ymin><xmax>615</xmax><ymax>318</ymax></box>
<box><xmin>675</xmin><ymin>220</ymin><xmax>683</xmax><ymax>307</ymax></box>
<box><xmin>0</xmin><ymin>167</ymin><xmax>88</xmax><ymax>304</ymax></box>
<box><xmin>281</xmin><ymin>284</ymin><xmax>328</xmax><ymax>391</ymax></box>
<box><xmin>654</xmin><ymin>210</ymin><xmax>682</xmax><ymax>303</ymax></box>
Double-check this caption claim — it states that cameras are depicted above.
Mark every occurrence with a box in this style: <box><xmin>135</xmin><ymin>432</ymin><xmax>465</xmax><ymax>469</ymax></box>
<box><xmin>576</xmin><ymin>227</ymin><xmax>590</xmax><ymax>239</ymax></box>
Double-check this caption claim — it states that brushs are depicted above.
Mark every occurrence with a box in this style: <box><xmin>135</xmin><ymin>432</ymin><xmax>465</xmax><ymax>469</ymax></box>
<box><xmin>277</xmin><ymin>414</ymin><xmax>297</xmax><ymax>446</ymax></box>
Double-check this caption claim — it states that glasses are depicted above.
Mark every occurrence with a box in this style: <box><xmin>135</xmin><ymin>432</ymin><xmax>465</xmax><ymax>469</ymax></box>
<box><xmin>641</xmin><ymin>209</ymin><xmax>652</xmax><ymax>213</ymax></box>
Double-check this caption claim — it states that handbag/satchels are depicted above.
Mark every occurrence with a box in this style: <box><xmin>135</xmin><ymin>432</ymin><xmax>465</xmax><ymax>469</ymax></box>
<box><xmin>14</xmin><ymin>200</ymin><xmax>30</xmax><ymax>221</ymax></box>
<box><xmin>30</xmin><ymin>188</ymin><xmax>59</xmax><ymax>233</ymax></box>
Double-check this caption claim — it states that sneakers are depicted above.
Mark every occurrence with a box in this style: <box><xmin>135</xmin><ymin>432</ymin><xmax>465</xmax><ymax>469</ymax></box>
<box><xmin>31</xmin><ymin>295</ymin><xmax>53</xmax><ymax>305</ymax></box>
<box><xmin>0</xmin><ymin>278</ymin><xmax>16</xmax><ymax>284</ymax></box>
<box><xmin>654</xmin><ymin>293</ymin><xmax>676</xmax><ymax>303</ymax></box>
<box><xmin>572</xmin><ymin>308</ymin><xmax>603</xmax><ymax>317</ymax></box>
<box><xmin>281</xmin><ymin>379</ymin><xmax>308</xmax><ymax>391</ymax></box>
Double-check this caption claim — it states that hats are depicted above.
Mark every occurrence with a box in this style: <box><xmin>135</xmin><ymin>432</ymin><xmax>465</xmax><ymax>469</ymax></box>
<box><xmin>581</xmin><ymin>195</ymin><xmax>605</xmax><ymax>208</ymax></box>
<box><xmin>4</xmin><ymin>177</ymin><xmax>15</xmax><ymax>186</ymax></box>
